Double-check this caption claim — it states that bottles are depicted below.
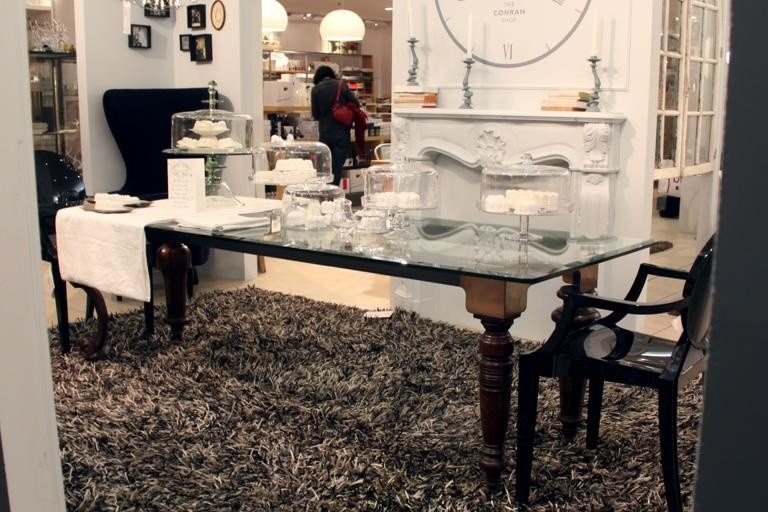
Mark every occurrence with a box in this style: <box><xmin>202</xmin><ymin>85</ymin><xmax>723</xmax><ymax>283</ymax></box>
<box><xmin>331</xmin><ymin>198</ymin><xmax>357</xmax><ymax>235</ymax></box>
<box><xmin>48</xmin><ymin>23</ymin><xmax>71</xmax><ymax>54</ymax></box>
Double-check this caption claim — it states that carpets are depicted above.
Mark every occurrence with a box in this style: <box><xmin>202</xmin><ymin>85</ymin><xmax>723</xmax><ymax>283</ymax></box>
<box><xmin>47</xmin><ymin>282</ymin><xmax>703</xmax><ymax>511</ymax></box>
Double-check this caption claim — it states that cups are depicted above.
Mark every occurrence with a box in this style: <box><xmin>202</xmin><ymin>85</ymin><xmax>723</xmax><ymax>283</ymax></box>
<box><xmin>29</xmin><ymin>21</ymin><xmax>48</xmax><ymax>52</ymax></box>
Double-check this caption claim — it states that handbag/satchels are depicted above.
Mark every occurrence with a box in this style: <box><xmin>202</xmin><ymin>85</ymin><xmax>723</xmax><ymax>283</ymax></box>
<box><xmin>333</xmin><ymin>104</ymin><xmax>354</xmax><ymax>127</ymax></box>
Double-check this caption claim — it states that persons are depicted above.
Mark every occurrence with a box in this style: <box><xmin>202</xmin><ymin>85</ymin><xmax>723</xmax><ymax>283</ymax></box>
<box><xmin>136</xmin><ymin>26</ymin><xmax>148</xmax><ymax>47</ymax></box>
<box><xmin>310</xmin><ymin>63</ymin><xmax>363</xmax><ymax>190</ymax></box>
<box><xmin>192</xmin><ymin>9</ymin><xmax>200</xmax><ymax>24</ymax></box>
<box><xmin>195</xmin><ymin>38</ymin><xmax>205</xmax><ymax>58</ymax></box>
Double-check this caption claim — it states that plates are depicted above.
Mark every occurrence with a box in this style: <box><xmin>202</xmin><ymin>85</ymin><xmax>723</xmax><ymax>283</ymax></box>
<box><xmin>86</xmin><ymin>197</ymin><xmax>154</xmax><ymax>208</ymax></box>
<box><xmin>80</xmin><ymin>203</ymin><xmax>134</xmax><ymax>214</ymax></box>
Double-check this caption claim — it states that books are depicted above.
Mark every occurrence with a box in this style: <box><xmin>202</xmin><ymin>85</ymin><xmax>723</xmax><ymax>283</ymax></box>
<box><xmin>539</xmin><ymin>89</ymin><xmax>592</xmax><ymax>113</ymax></box>
<box><xmin>393</xmin><ymin>85</ymin><xmax>440</xmax><ymax>110</ymax></box>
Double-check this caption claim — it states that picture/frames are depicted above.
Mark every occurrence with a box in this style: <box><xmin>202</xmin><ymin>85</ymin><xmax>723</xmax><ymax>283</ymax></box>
<box><xmin>180</xmin><ymin>34</ymin><xmax>192</xmax><ymax>52</ymax></box>
<box><xmin>186</xmin><ymin>3</ymin><xmax>206</xmax><ymax>28</ymax></box>
<box><xmin>128</xmin><ymin>24</ymin><xmax>152</xmax><ymax>49</ymax></box>
<box><xmin>189</xmin><ymin>34</ymin><xmax>212</xmax><ymax>61</ymax></box>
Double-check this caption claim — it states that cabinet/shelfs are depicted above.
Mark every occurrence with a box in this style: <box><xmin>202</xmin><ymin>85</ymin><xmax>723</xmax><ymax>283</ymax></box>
<box><xmin>262</xmin><ymin>46</ymin><xmax>390</xmax><ymax>195</ymax></box>
<box><xmin>28</xmin><ymin>51</ymin><xmax>80</xmax><ymax>156</ymax></box>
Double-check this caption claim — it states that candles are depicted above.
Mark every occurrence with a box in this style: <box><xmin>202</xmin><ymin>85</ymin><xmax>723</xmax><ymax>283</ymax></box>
<box><xmin>434</xmin><ymin>0</ymin><xmax>593</xmax><ymax>69</ymax></box>
<box><xmin>592</xmin><ymin>8</ymin><xmax>599</xmax><ymax>59</ymax></box>
<box><xmin>405</xmin><ymin>1</ymin><xmax>415</xmax><ymax>39</ymax></box>
<box><xmin>466</xmin><ymin>12</ymin><xmax>474</xmax><ymax>60</ymax></box>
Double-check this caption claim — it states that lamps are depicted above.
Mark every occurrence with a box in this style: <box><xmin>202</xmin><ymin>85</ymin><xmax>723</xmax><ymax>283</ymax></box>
<box><xmin>127</xmin><ymin>0</ymin><xmax>196</xmax><ymax>14</ymax></box>
<box><xmin>319</xmin><ymin>0</ymin><xmax>366</xmax><ymax>42</ymax></box>
<box><xmin>261</xmin><ymin>0</ymin><xmax>287</xmax><ymax>32</ymax></box>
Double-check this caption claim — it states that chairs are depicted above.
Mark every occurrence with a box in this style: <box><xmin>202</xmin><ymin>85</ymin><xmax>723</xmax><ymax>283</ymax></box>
<box><xmin>35</xmin><ymin>150</ymin><xmax>155</xmax><ymax>354</ymax></box>
<box><xmin>510</xmin><ymin>230</ymin><xmax>716</xmax><ymax>507</ymax></box>
<box><xmin>97</xmin><ymin>86</ymin><xmax>219</xmax><ymax>303</ymax></box>
